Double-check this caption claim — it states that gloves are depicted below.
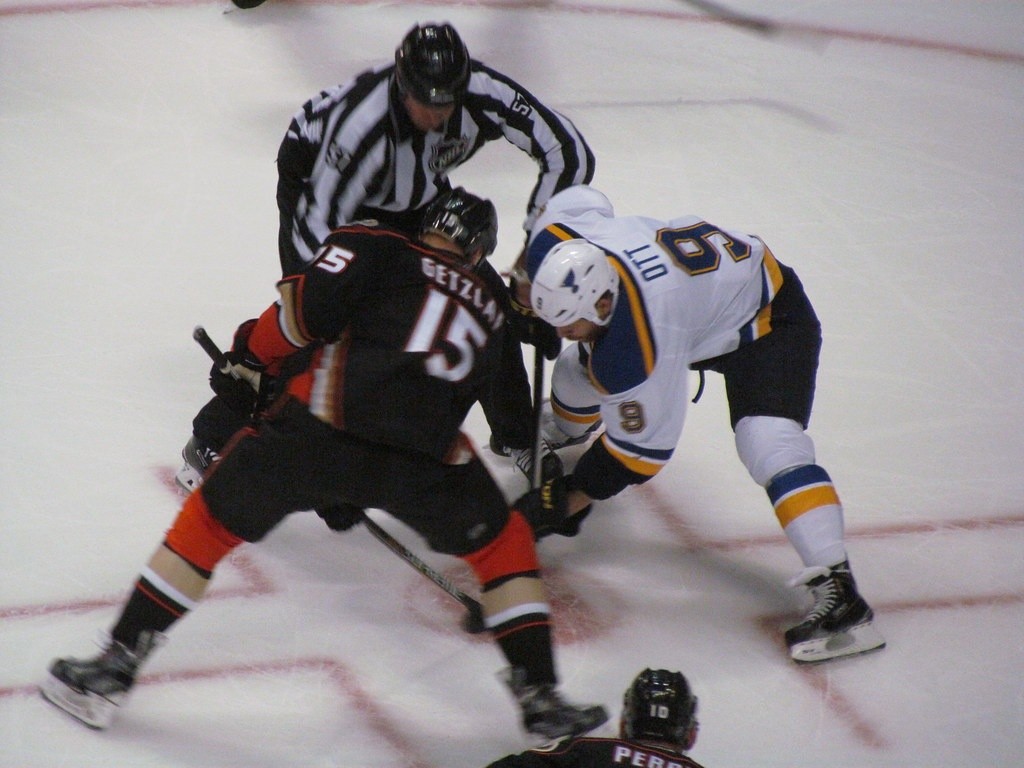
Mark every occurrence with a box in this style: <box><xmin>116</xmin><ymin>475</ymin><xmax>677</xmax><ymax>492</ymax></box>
<box><xmin>508</xmin><ymin>475</ymin><xmax>593</xmax><ymax>539</ymax></box>
<box><xmin>211</xmin><ymin>319</ymin><xmax>276</xmax><ymax>409</ymax></box>
<box><xmin>508</xmin><ymin>295</ymin><xmax>562</xmax><ymax>358</ymax></box>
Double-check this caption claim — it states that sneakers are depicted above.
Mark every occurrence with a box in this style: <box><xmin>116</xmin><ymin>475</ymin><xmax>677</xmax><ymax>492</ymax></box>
<box><xmin>505</xmin><ymin>435</ymin><xmax>562</xmax><ymax>483</ymax></box>
<box><xmin>782</xmin><ymin>564</ymin><xmax>887</xmax><ymax>662</ymax></box>
<box><xmin>41</xmin><ymin>642</ymin><xmax>140</xmax><ymax>730</ymax></box>
<box><xmin>490</xmin><ymin>411</ymin><xmax>590</xmax><ymax>454</ymax></box>
<box><xmin>174</xmin><ymin>432</ymin><xmax>220</xmax><ymax>493</ymax></box>
<box><xmin>500</xmin><ymin>669</ymin><xmax>608</xmax><ymax>746</ymax></box>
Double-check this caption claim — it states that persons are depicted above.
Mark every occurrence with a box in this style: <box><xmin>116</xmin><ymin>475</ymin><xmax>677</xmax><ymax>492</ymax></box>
<box><xmin>480</xmin><ymin>666</ymin><xmax>710</xmax><ymax>768</ymax></box>
<box><xmin>494</xmin><ymin>185</ymin><xmax>886</xmax><ymax>662</ymax></box>
<box><xmin>173</xmin><ymin>19</ymin><xmax>596</xmax><ymax>498</ymax></box>
<box><xmin>34</xmin><ymin>183</ymin><xmax>610</xmax><ymax>748</ymax></box>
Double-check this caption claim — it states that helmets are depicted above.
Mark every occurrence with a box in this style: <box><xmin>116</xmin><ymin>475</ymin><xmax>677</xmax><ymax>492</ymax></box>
<box><xmin>394</xmin><ymin>23</ymin><xmax>471</xmax><ymax>107</ymax></box>
<box><xmin>618</xmin><ymin>669</ymin><xmax>697</xmax><ymax>749</ymax></box>
<box><xmin>532</xmin><ymin>239</ymin><xmax>619</xmax><ymax>327</ymax></box>
<box><xmin>419</xmin><ymin>187</ymin><xmax>498</xmax><ymax>272</ymax></box>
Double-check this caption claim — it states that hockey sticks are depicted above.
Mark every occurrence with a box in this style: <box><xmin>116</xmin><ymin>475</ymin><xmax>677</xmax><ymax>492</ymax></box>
<box><xmin>530</xmin><ymin>347</ymin><xmax>545</xmax><ymax>488</ymax></box>
<box><xmin>195</xmin><ymin>331</ymin><xmax>485</xmax><ymax>635</ymax></box>
